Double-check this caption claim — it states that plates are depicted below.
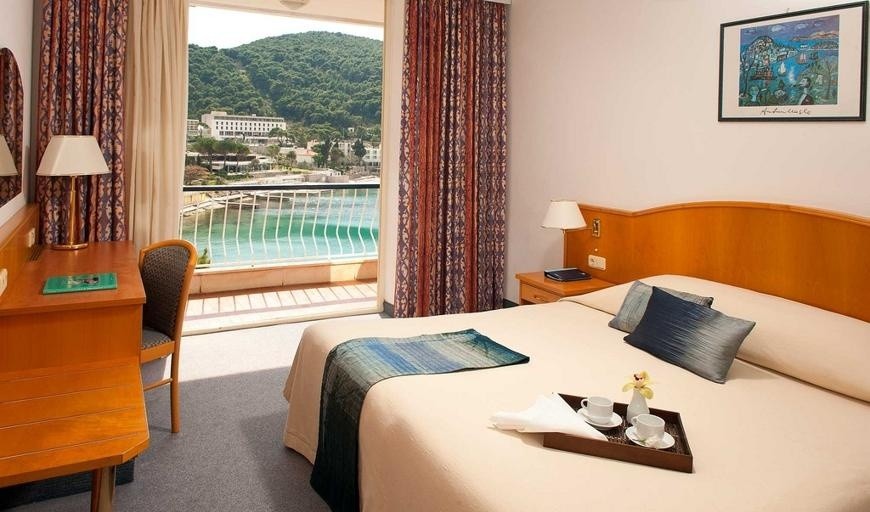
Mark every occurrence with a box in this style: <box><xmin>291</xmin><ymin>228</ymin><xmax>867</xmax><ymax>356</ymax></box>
<box><xmin>625</xmin><ymin>426</ymin><xmax>675</xmax><ymax>449</ymax></box>
<box><xmin>577</xmin><ymin>408</ymin><xmax>622</xmax><ymax>431</ymax></box>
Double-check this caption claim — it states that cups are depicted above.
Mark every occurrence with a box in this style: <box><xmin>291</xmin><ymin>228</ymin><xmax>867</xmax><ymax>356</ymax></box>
<box><xmin>631</xmin><ymin>414</ymin><xmax>665</xmax><ymax>440</ymax></box>
<box><xmin>580</xmin><ymin>396</ymin><xmax>613</xmax><ymax>425</ymax></box>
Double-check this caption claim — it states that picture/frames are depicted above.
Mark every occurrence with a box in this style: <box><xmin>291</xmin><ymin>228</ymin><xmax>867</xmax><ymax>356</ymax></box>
<box><xmin>718</xmin><ymin>2</ymin><xmax>868</xmax><ymax>121</ymax></box>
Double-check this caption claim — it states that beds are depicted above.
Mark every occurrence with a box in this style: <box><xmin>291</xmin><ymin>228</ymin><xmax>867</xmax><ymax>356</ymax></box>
<box><xmin>306</xmin><ymin>273</ymin><xmax>870</xmax><ymax>512</ymax></box>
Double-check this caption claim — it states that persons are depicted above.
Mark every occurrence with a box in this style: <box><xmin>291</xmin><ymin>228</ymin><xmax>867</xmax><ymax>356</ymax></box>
<box><xmin>798</xmin><ymin>77</ymin><xmax>814</xmax><ymax>105</ymax></box>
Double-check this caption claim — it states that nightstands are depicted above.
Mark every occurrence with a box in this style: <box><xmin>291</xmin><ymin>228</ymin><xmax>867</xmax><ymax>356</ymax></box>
<box><xmin>515</xmin><ymin>269</ymin><xmax>615</xmax><ymax>306</ymax></box>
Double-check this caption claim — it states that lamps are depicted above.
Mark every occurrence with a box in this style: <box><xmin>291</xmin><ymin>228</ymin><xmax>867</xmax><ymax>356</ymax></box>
<box><xmin>541</xmin><ymin>201</ymin><xmax>592</xmax><ymax>281</ymax></box>
<box><xmin>35</xmin><ymin>135</ymin><xmax>107</xmax><ymax>249</ymax></box>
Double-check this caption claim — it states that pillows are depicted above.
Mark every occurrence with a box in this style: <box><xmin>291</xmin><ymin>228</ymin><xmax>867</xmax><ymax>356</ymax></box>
<box><xmin>623</xmin><ymin>286</ymin><xmax>753</xmax><ymax>383</ymax></box>
<box><xmin>609</xmin><ymin>279</ymin><xmax>713</xmax><ymax>335</ymax></box>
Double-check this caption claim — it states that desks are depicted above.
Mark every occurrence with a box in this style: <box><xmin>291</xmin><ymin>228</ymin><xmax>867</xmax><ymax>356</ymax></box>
<box><xmin>1</xmin><ymin>203</ymin><xmax>146</xmax><ymax>510</ymax></box>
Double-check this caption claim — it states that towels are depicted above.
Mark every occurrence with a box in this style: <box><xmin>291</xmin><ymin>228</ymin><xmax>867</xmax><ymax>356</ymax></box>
<box><xmin>487</xmin><ymin>391</ymin><xmax>609</xmax><ymax>445</ymax></box>
<box><xmin>310</xmin><ymin>329</ymin><xmax>529</xmax><ymax>512</ymax></box>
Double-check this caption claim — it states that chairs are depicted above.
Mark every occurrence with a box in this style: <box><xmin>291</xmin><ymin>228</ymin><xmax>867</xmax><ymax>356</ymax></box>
<box><xmin>139</xmin><ymin>240</ymin><xmax>196</xmax><ymax>433</ymax></box>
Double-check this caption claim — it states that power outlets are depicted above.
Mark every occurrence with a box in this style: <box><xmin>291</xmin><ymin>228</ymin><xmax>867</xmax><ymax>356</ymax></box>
<box><xmin>588</xmin><ymin>254</ymin><xmax>605</xmax><ymax>270</ymax></box>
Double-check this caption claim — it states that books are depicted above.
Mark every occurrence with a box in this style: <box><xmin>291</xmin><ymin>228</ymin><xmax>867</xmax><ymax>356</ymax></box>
<box><xmin>41</xmin><ymin>272</ymin><xmax>120</xmax><ymax>294</ymax></box>
